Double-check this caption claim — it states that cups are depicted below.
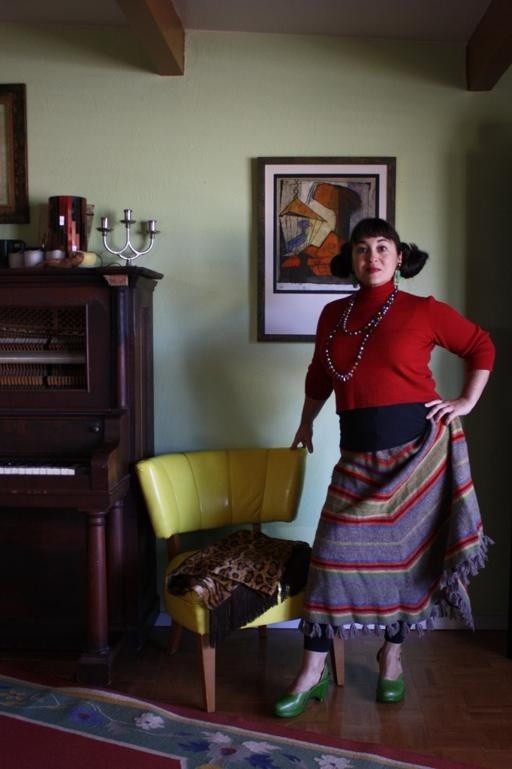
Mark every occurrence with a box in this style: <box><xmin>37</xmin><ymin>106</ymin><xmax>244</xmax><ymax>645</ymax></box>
<box><xmin>48</xmin><ymin>195</ymin><xmax>89</xmax><ymax>254</ymax></box>
<box><xmin>0</xmin><ymin>238</ymin><xmax>97</xmax><ymax>267</ymax></box>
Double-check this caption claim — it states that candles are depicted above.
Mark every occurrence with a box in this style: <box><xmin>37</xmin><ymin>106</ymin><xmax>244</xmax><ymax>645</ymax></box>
<box><xmin>101</xmin><ymin>216</ymin><xmax>108</xmax><ymax>227</ymax></box>
<box><xmin>123</xmin><ymin>207</ymin><xmax>133</xmax><ymax>220</ymax></box>
<box><xmin>148</xmin><ymin>218</ymin><xmax>158</xmax><ymax>230</ymax></box>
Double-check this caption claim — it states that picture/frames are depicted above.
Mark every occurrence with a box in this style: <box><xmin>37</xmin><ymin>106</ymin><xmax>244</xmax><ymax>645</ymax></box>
<box><xmin>252</xmin><ymin>154</ymin><xmax>398</xmax><ymax>343</ymax></box>
<box><xmin>0</xmin><ymin>80</ymin><xmax>32</xmax><ymax>226</ymax></box>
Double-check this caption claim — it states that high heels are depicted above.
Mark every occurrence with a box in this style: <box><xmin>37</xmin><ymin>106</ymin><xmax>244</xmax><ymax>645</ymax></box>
<box><xmin>275</xmin><ymin>660</ymin><xmax>331</xmax><ymax>718</ymax></box>
<box><xmin>376</xmin><ymin>646</ymin><xmax>404</xmax><ymax>702</ymax></box>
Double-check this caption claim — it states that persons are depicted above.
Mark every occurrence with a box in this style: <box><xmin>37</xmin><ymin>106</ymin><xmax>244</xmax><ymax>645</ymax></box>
<box><xmin>273</xmin><ymin>218</ymin><xmax>498</xmax><ymax>719</ymax></box>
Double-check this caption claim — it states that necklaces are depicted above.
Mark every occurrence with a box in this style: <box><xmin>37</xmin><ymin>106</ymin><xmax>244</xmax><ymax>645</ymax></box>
<box><xmin>325</xmin><ymin>285</ymin><xmax>398</xmax><ymax>382</ymax></box>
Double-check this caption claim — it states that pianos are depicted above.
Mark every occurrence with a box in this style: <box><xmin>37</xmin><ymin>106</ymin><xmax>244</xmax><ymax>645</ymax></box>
<box><xmin>0</xmin><ymin>264</ymin><xmax>161</xmax><ymax>661</ymax></box>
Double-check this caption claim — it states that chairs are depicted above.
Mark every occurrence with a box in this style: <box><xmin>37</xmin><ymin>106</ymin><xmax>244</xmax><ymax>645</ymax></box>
<box><xmin>132</xmin><ymin>444</ymin><xmax>347</xmax><ymax>714</ymax></box>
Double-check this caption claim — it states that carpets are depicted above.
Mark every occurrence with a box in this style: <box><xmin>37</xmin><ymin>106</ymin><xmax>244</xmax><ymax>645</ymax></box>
<box><xmin>0</xmin><ymin>660</ymin><xmax>488</xmax><ymax>769</ymax></box>
